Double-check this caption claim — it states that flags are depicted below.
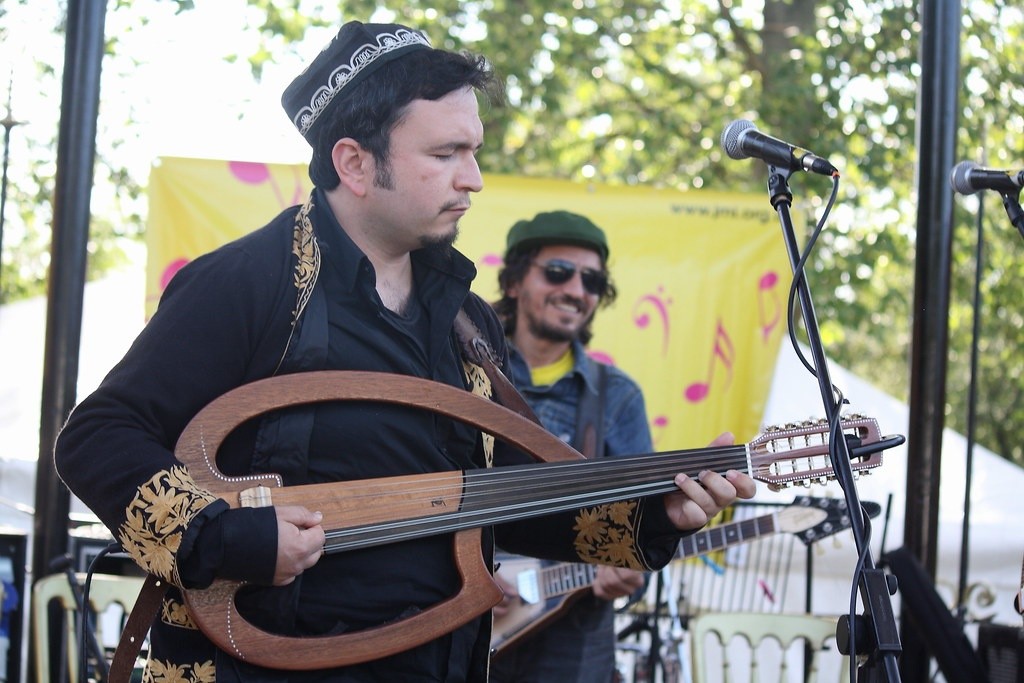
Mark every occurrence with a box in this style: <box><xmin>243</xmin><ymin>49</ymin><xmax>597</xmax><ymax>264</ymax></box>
<box><xmin>148</xmin><ymin>156</ymin><xmax>804</xmax><ymax>572</ymax></box>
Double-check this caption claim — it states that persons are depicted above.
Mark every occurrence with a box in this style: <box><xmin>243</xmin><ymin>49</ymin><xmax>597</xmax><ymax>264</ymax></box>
<box><xmin>54</xmin><ymin>21</ymin><xmax>754</xmax><ymax>683</ymax></box>
<box><xmin>488</xmin><ymin>212</ymin><xmax>659</xmax><ymax>683</ymax></box>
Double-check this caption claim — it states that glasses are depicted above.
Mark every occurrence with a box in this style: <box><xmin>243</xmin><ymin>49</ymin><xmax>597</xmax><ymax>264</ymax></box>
<box><xmin>531</xmin><ymin>259</ymin><xmax>605</xmax><ymax>295</ymax></box>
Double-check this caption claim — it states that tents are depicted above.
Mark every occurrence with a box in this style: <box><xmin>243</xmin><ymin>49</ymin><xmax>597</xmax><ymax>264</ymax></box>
<box><xmin>0</xmin><ymin>260</ymin><xmax>1024</xmax><ymax>683</ymax></box>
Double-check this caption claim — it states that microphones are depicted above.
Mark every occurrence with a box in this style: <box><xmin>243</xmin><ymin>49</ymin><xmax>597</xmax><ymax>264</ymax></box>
<box><xmin>948</xmin><ymin>158</ymin><xmax>1024</xmax><ymax>196</ymax></box>
<box><xmin>722</xmin><ymin>120</ymin><xmax>839</xmax><ymax>179</ymax></box>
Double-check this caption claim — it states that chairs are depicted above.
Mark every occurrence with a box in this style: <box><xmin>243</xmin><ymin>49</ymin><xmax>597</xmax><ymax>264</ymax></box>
<box><xmin>688</xmin><ymin>612</ymin><xmax>850</xmax><ymax>683</ymax></box>
<box><xmin>33</xmin><ymin>573</ymin><xmax>145</xmax><ymax>683</ymax></box>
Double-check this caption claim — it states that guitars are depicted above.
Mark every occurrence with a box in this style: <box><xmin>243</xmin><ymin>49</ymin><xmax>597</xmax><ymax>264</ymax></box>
<box><xmin>489</xmin><ymin>488</ymin><xmax>881</xmax><ymax>658</ymax></box>
<box><xmin>175</xmin><ymin>370</ymin><xmax>884</xmax><ymax>672</ymax></box>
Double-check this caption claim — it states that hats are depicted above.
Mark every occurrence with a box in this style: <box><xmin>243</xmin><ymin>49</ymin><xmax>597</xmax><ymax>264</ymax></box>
<box><xmin>281</xmin><ymin>20</ymin><xmax>433</xmax><ymax>147</ymax></box>
<box><xmin>504</xmin><ymin>210</ymin><xmax>609</xmax><ymax>264</ymax></box>
